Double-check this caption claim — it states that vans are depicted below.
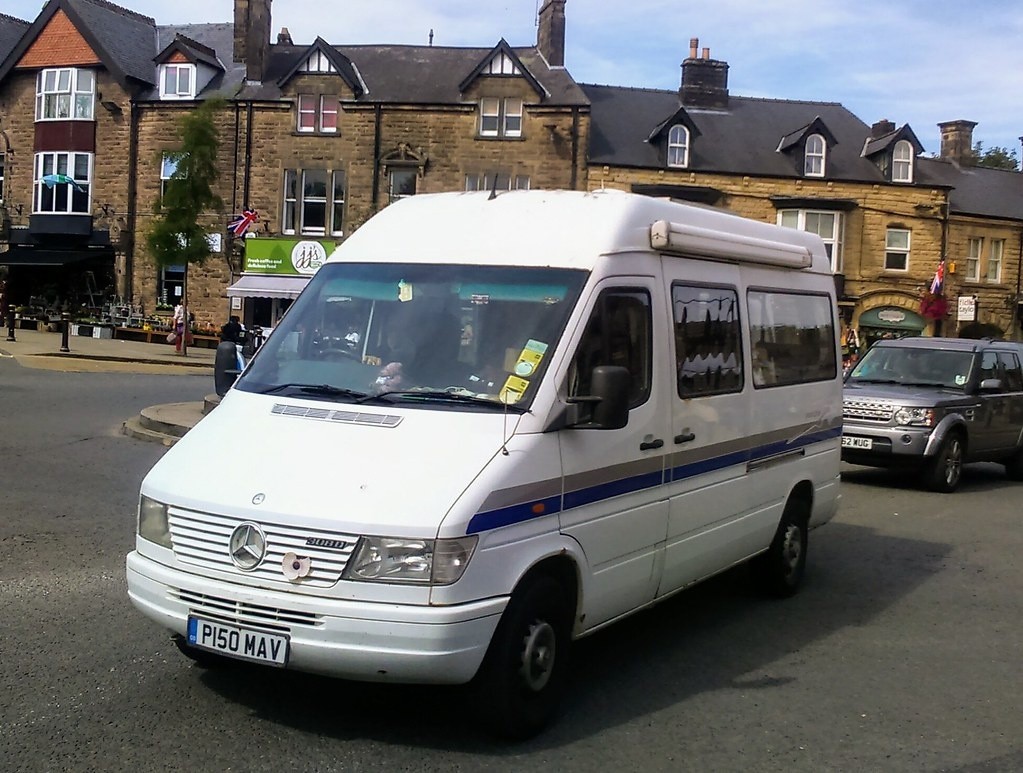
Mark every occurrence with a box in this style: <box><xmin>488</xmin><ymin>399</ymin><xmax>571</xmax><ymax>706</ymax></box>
<box><xmin>124</xmin><ymin>188</ymin><xmax>844</xmax><ymax>728</ymax></box>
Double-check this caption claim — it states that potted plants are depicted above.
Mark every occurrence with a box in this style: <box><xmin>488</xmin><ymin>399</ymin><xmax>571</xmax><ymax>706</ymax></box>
<box><xmin>73</xmin><ymin>317</ymin><xmax>98</xmax><ymax>337</ymax></box>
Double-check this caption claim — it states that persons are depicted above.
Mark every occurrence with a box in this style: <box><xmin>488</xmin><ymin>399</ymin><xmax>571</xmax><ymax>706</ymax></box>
<box><xmin>220</xmin><ymin>316</ymin><xmax>246</xmax><ymax>345</ymax></box>
<box><xmin>0</xmin><ymin>293</ymin><xmax>6</xmax><ymax>327</ymax></box>
<box><xmin>361</xmin><ymin>297</ymin><xmax>448</xmax><ymax>385</ymax></box>
<box><xmin>297</xmin><ymin>319</ymin><xmax>360</xmax><ymax>359</ymax></box>
<box><xmin>172</xmin><ymin>298</ymin><xmax>190</xmax><ymax>353</ymax></box>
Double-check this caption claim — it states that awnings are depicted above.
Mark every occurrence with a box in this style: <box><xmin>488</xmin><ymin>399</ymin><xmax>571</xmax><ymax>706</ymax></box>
<box><xmin>0</xmin><ymin>248</ymin><xmax>108</xmax><ymax>265</ymax></box>
<box><xmin>225</xmin><ymin>272</ymin><xmax>314</xmax><ymax>300</ymax></box>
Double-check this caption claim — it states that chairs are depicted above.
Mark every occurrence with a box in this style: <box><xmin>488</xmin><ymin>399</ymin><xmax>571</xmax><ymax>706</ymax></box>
<box><xmin>357</xmin><ymin>299</ymin><xmax>579</xmax><ymax>403</ymax></box>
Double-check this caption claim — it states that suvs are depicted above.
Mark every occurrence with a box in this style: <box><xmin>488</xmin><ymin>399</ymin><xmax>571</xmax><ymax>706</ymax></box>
<box><xmin>842</xmin><ymin>334</ymin><xmax>1023</xmax><ymax>492</ymax></box>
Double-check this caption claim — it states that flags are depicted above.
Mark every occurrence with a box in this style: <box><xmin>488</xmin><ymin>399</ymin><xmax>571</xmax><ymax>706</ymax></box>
<box><xmin>930</xmin><ymin>255</ymin><xmax>947</xmax><ymax>296</ymax></box>
<box><xmin>226</xmin><ymin>208</ymin><xmax>261</xmax><ymax>237</ymax></box>
